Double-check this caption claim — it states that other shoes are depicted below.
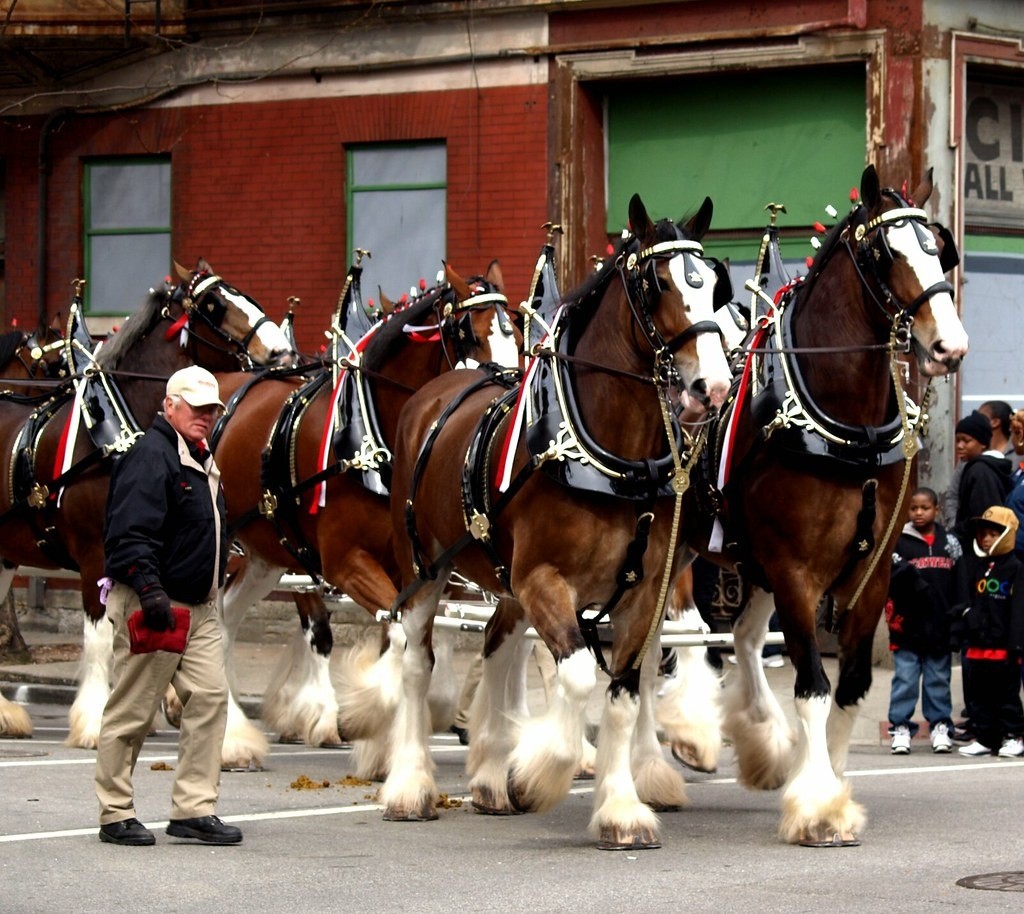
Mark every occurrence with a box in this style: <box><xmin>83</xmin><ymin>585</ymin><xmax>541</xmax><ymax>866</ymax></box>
<box><xmin>955</xmin><ymin>721</ymin><xmax>969</xmax><ymax>733</ymax></box>
<box><xmin>953</xmin><ymin>732</ymin><xmax>976</xmax><ymax>746</ymax></box>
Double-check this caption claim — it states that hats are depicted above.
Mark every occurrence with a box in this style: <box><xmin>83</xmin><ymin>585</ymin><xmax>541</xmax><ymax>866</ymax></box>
<box><xmin>166</xmin><ymin>365</ymin><xmax>226</xmax><ymax>411</ymax></box>
<box><xmin>954</xmin><ymin>410</ymin><xmax>993</xmax><ymax>451</ymax></box>
<box><xmin>970</xmin><ymin>505</ymin><xmax>1020</xmax><ymax>557</ymax></box>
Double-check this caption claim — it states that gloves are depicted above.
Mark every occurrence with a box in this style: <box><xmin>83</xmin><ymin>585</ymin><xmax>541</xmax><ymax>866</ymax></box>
<box><xmin>139</xmin><ymin>583</ymin><xmax>176</xmax><ymax>631</ymax></box>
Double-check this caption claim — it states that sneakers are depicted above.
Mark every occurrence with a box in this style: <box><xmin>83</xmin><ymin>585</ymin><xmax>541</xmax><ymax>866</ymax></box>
<box><xmin>165</xmin><ymin>815</ymin><xmax>243</xmax><ymax>843</ymax></box>
<box><xmin>98</xmin><ymin>818</ymin><xmax>155</xmax><ymax>846</ymax></box>
<box><xmin>889</xmin><ymin>725</ymin><xmax>912</xmax><ymax>754</ymax></box>
<box><xmin>930</xmin><ymin>722</ymin><xmax>953</xmax><ymax>752</ymax></box>
<box><xmin>998</xmin><ymin>738</ymin><xmax>1024</xmax><ymax>758</ymax></box>
<box><xmin>957</xmin><ymin>741</ymin><xmax>992</xmax><ymax>757</ymax></box>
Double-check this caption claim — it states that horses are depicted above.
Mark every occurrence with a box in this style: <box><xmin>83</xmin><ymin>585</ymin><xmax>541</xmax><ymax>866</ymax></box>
<box><xmin>1</xmin><ymin>165</ymin><xmax>971</xmax><ymax>852</ymax></box>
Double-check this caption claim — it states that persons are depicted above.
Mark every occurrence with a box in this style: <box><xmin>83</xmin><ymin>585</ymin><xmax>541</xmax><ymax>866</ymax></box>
<box><xmin>885</xmin><ymin>400</ymin><xmax>1024</xmax><ymax>757</ymax></box>
<box><xmin>94</xmin><ymin>365</ymin><xmax>242</xmax><ymax>843</ymax></box>
